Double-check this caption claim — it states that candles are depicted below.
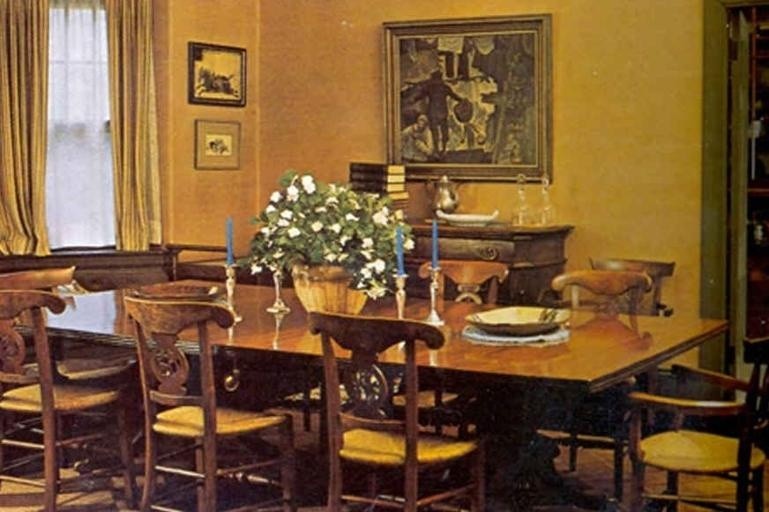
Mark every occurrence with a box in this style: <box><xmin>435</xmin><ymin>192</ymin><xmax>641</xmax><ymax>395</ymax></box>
<box><xmin>226</xmin><ymin>215</ymin><xmax>234</xmax><ymax>266</ymax></box>
<box><xmin>394</xmin><ymin>226</ymin><xmax>407</xmax><ymax>272</ymax></box>
<box><xmin>431</xmin><ymin>218</ymin><xmax>438</xmax><ymax>271</ymax></box>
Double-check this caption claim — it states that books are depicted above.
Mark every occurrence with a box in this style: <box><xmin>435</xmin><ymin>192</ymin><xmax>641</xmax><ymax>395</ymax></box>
<box><xmin>350</xmin><ymin>163</ymin><xmax>405</xmax><ymax>175</ymax></box>
<box><xmin>351</xmin><ymin>182</ymin><xmax>406</xmax><ymax>192</ymax></box>
<box><xmin>350</xmin><ymin>172</ymin><xmax>406</xmax><ymax>183</ymax></box>
<box><xmin>384</xmin><ymin>193</ymin><xmax>409</xmax><ymax>200</ymax></box>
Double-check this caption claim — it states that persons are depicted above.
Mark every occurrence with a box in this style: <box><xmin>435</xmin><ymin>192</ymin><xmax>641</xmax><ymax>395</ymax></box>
<box><xmin>403</xmin><ymin>115</ymin><xmax>434</xmax><ymax>162</ymax></box>
<box><xmin>410</xmin><ymin>70</ymin><xmax>463</xmax><ymax>156</ymax></box>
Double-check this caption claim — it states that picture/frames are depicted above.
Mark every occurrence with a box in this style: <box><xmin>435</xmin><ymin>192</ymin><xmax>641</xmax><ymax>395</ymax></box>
<box><xmin>194</xmin><ymin>120</ymin><xmax>242</xmax><ymax>171</ymax></box>
<box><xmin>381</xmin><ymin>11</ymin><xmax>555</xmax><ymax>186</ymax></box>
<box><xmin>187</xmin><ymin>40</ymin><xmax>248</xmax><ymax>105</ymax></box>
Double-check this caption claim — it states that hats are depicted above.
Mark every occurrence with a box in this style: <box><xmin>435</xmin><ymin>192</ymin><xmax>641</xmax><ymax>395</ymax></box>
<box><xmin>427</xmin><ymin>68</ymin><xmax>441</xmax><ymax>79</ymax></box>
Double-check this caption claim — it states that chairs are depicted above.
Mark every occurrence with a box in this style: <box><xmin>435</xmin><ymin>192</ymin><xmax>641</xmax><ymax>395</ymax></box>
<box><xmin>627</xmin><ymin>336</ymin><xmax>769</xmax><ymax>512</ymax></box>
<box><xmin>0</xmin><ymin>266</ymin><xmax>77</xmax><ymax>294</ymax></box>
<box><xmin>308</xmin><ymin>312</ymin><xmax>486</xmax><ymax>511</ymax></box>
<box><xmin>588</xmin><ymin>255</ymin><xmax>676</xmax><ymax>315</ymax></box>
<box><xmin>286</xmin><ymin>380</ymin><xmax>349</xmax><ymax>406</ymax></box>
<box><xmin>548</xmin><ymin>272</ymin><xmax>655</xmax><ymax>469</ymax></box>
<box><xmin>124</xmin><ymin>297</ymin><xmax>298</xmax><ymax>511</ymax></box>
<box><xmin>393</xmin><ymin>261</ymin><xmax>510</xmax><ymax>424</ymax></box>
<box><xmin>0</xmin><ymin>290</ymin><xmax>137</xmax><ymax>511</ymax></box>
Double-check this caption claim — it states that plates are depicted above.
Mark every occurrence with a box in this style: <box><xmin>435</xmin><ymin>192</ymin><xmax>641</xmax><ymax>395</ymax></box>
<box><xmin>436</xmin><ymin>208</ymin><xmax>500</xmax><ymax>227</ymax></box>
<box><xmin>465</xmin><ymin>304</ymin><xmax>571</xmax><ymax>337</ymax></box>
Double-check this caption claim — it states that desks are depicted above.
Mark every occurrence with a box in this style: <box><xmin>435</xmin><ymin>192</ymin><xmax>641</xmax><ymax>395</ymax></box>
<box><xmin>390</xmin><ymin>223</ymin><xmax>573</xmax><ymax>304</ymax></box>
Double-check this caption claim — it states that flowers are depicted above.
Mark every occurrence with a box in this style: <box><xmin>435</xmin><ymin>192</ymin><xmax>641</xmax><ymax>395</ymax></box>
<box><xmin>237</xmin><ymin>170</ymin><xmax>414</xmax><ymax>299</ymax></box>
